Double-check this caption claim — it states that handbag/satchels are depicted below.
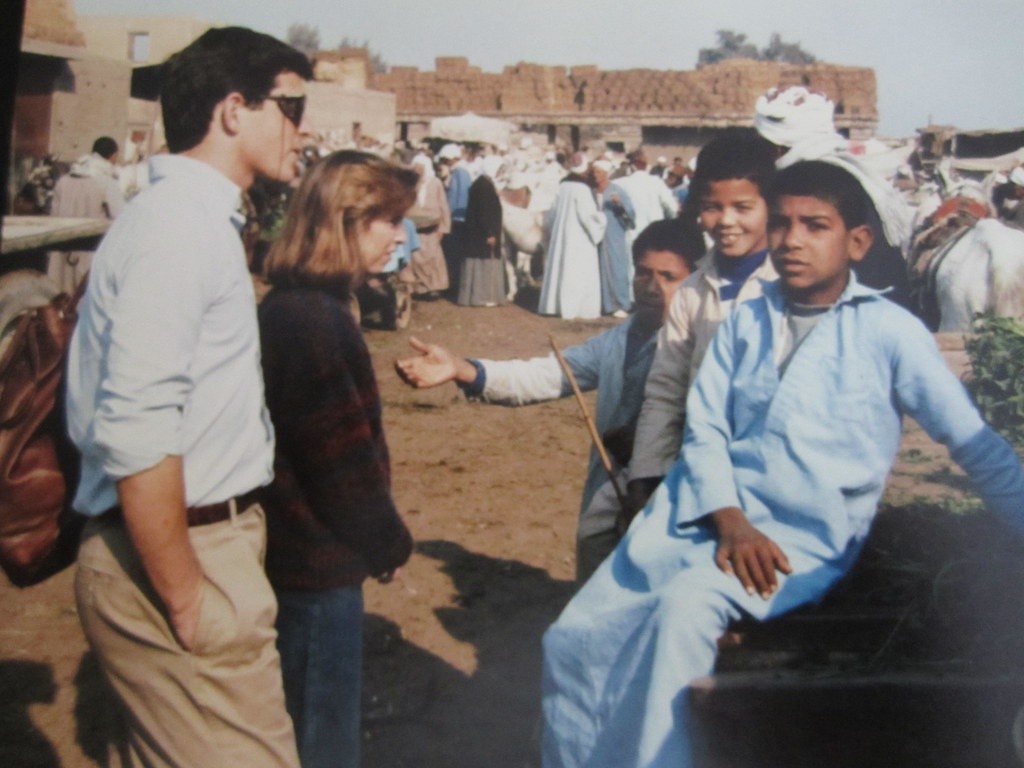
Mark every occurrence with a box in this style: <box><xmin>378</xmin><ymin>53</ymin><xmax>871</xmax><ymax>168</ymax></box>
<box><xmin>0</xmin><ymin>269</ymin><xmax>91</xmax><ymax>589</ymax></box>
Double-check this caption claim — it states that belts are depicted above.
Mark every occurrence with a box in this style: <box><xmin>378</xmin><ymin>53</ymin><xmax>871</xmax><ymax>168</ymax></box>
<box><xmin>96</xmin><ymin>480</ymin><xmax>264</xmax><ymax>528</ymax></box>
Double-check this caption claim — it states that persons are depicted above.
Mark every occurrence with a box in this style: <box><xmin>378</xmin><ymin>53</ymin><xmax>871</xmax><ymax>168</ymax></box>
<box><xmin>70</xmin><ymin>24</ymin><xmax>321</xmax><ymax>767</ymax></box>
<box><xmin>624</xmin><ymin>126</ymin><xmax>781</xmax><ymax>538</ymax></box>
<box><xmin>64</xmin><ymin>90</ymin><xmax>1022</xmax><ymax>319</ymax></box>
<box><xmin>392</xmin><ymin>214</ymin><xmax>707</xmax><ymax>588</ymax></box>
<box><xmin>251</xmin><ymin>144</ymin><xmax>420</xmax><ymax>767</ymax></box>
<box><xmin>534</xmin><ymin>144</ymin><xmax>1022</xmax><ymax>768</ymax></box>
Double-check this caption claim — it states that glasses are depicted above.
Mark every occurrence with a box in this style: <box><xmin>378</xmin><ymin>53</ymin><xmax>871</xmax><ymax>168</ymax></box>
<box><xmin>243</xmin><ymin>93</ymin><xmax>306</xmax><ymax>128</ymax></box>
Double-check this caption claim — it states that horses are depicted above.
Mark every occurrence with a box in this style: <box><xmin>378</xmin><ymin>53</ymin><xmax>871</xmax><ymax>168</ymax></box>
<box><xmin>903</xmin><ymin>181</ymin><xmax>1023</xmax><ymax>334</ymax></box>
